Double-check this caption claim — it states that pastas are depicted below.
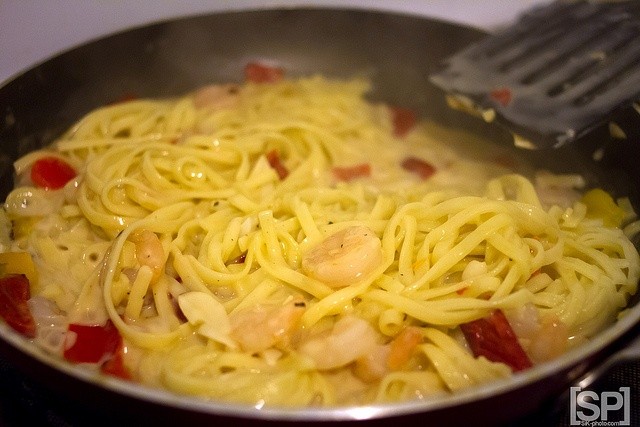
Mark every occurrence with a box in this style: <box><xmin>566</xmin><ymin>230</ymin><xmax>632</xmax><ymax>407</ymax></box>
<box><xmin>0</xmin><ymin>76</ymin><xmax>639</xmax><ymax>406</ymax></box>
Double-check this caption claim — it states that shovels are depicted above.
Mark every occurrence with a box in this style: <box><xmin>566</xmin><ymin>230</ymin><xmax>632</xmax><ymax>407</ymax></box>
<box><xmin>427</xmin><ymin>0</ymin><xmax>640</xmax><ymax>149</ymax></box>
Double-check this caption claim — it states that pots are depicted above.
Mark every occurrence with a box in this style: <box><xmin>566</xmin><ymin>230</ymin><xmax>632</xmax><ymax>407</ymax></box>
<box><xmin>0</xmin><ymin>8</ymin><xmax>640</xmax><ymax>427</ymax></box>
